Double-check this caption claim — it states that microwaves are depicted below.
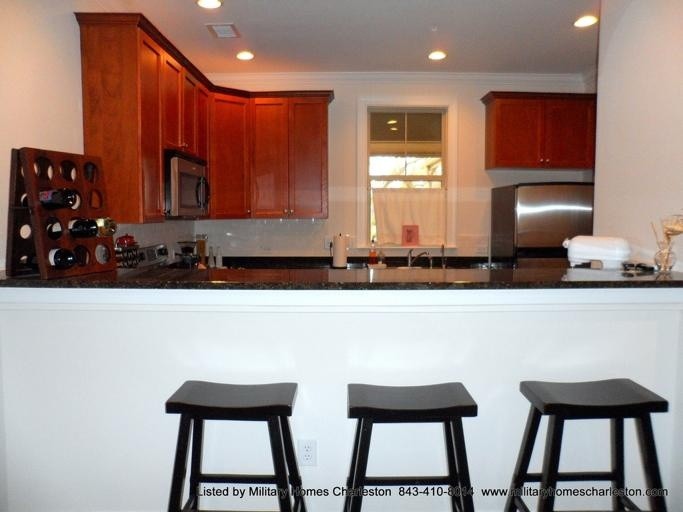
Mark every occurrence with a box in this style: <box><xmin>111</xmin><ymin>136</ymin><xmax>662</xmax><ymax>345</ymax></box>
<box><xmin>163</xmin><ymin>149</ymin><xmax>210</xmax><ymax>218</ymax></box>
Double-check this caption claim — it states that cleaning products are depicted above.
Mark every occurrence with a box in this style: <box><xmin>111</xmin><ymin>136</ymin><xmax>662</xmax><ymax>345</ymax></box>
<box><xmin>370</xmin><ymin>240</ymin><xmax>378</xmax><ymax>264</ymax></box>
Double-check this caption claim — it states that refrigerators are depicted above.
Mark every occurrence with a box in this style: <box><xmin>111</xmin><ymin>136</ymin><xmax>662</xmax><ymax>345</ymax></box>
<box><xmin>490</xmin><ymin>181</ymin><xmax>594</xmax><ymax>270</ymax></box>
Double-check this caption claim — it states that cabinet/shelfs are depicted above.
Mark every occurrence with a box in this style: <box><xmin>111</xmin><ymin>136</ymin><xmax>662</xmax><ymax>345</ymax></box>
<box><xmin>484</xmin><ymin>99</ymin><xmax>594</xmax><ymax>169</ymax></box>
<box><xmin>80</xmin><ymin>26</ymin><xmax>164</xmax><ymax>224</ymax></box>
<box><xmin>194</xmin><ymin>86</ymin><xmax>208</xmax><ymax>158</ymax></box>
<box><xmin>206</xmin><ymin>97</ymin><xmax>250</xmax><ymax>221</ymax></box>
<box><xmin>165</xmin><ymin>57</ymin><xmax>195</xmax><ymax>154</ymax></box>
<box><xmin>251</xmin><ymin>96</ymin><xmax>327</xmax><ymax>219</ymax></box>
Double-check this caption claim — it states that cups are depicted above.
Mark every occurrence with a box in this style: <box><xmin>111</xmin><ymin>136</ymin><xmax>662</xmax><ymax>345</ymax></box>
<box><xmin>195</xmin><ymin>233</ymin><xmax>208</xmax><ymax>264</ymax></box>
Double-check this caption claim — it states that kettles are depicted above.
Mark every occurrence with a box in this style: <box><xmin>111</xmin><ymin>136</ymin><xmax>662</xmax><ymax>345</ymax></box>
<box><xmin>331</xmin><ymin>234</ymin><xmax>351</xmax><ymax>267</ymax></box>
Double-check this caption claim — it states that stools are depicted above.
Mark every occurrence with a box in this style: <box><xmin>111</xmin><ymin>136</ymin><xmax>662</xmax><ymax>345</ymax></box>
<box><xmin>163</xmin><ymin>378</ymin><xmax>309</xmax><ymax>511</ymax></box>
<box><xmin>502</xmin><ymin>377</ymin><xmax>669</xmax><ymax>510</ymax></box>
<box><xmin>343</xmin><ymin>380</ymin><xmax>478</xmax><ymax>512</ymax></box>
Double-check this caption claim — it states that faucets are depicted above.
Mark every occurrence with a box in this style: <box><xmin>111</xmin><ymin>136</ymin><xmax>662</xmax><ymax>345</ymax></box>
<box><xmin>407</xmin><ymin>247</ymin><xmax>430</xmax><ymax>267</ymax></box>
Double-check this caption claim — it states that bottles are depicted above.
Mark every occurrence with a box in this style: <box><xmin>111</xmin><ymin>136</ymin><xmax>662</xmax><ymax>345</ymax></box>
<box><xmin>22</xmin><ymin>189</ymin><xmax>77</xmax><ymax>209</ymax></box>
<box><xmin>207</xmin><ymin>246</ymin><xmax>215</xmax><ymax>267</ymax></box>
<box><xmin>215</xmin><ymin>246</ymin><xmax>222</xmax><ymax>267</ymax></box>
<box><xmin>95</xmin><ymin>217</ymin><xmax>116</xmax><ymax>237</ymax></box>
<box><xmin>368</xmin><ymin>239</ymin><xmax>376</xmax><ymax>264</ymax></box>
<box><xmin>47</xmin><ymin>219</ymin><xmax>98</xmax><ymax>239</ymax></box>
<box><xmin>25</xmin><ymin>247</ymin><xmax>74</xmax><ymax>268</ymax></box>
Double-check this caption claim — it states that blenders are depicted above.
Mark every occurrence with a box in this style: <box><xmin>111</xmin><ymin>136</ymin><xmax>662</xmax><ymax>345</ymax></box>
<box><xmin>177</xmin><ymin>240</ymin><xmax>196</xmax><ymax>256</ymax></box>
